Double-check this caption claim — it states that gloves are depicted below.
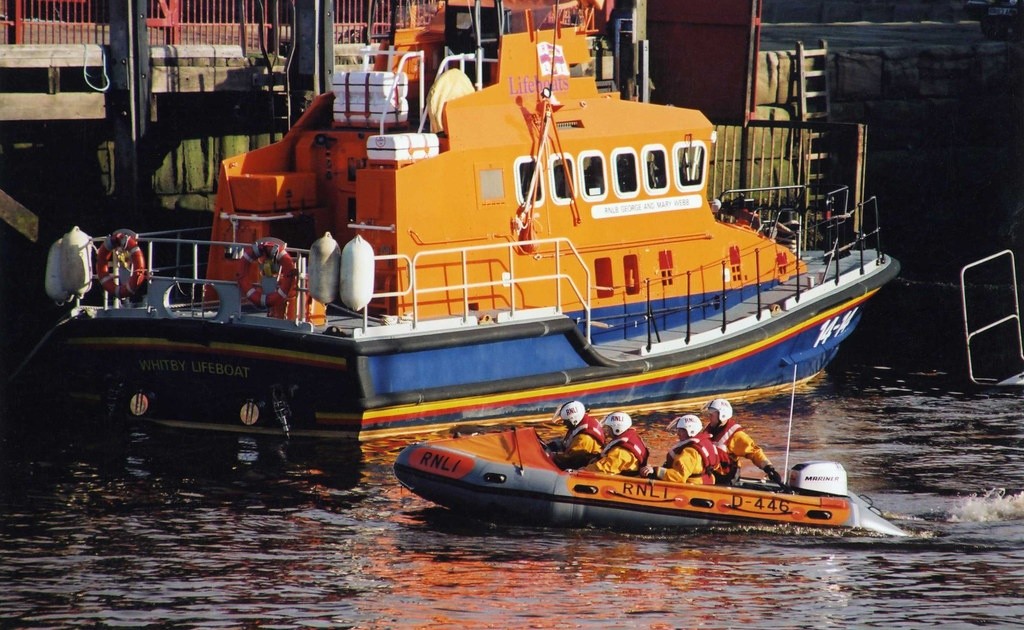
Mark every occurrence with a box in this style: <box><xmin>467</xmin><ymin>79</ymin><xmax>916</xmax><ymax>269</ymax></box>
<box><xmin>763</xmin><ymin>465</ymin><xmax>782</xmax><ymax>484</ymax></box>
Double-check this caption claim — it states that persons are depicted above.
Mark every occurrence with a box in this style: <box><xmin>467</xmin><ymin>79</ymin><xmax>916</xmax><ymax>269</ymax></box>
<box><xmin>641</xmin><ymin>414</ymin><xmax>719</xmax><ymax>484</ymax></box>
<box><xmin>703</xmin><ymin>398</ymin><xmax>781</xmax><ymax>485</ymax></box>
<box><xmin>565</xmin><ymin>412</ymin><xmax>650</xmax><ymax>474</ymax></box>
<box><xmin>545</xmin><ymin>400</ymin><xmax>605</xmax><ymax>469</ymax></box>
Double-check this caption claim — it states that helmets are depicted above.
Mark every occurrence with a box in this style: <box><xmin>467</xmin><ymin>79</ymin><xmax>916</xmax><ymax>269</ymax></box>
<box><xmin>677</xmin><ymin>414</ymin><xmax>702</xmax><ymax>437</ymax></box>
<box><xmin>604</xmin><ymin>411</ymin><xmax>632</xmax><ymax>436</ymax></box>
<box><xmin>709</xmin><ymin>399</ymin><xmax>733</xmax><ymax>422</ymax></box>
<box><xmin>560</xmin><ymin>400</ymin><xmax>586</xmax><ymax>427</ymax></box>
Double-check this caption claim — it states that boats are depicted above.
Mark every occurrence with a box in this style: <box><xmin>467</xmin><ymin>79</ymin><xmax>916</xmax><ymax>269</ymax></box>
<box><xmin>18</xmin><ymin>0</ymin><xmax>905</xmax><ymax>455</ymax></box>
<box><xmin>394</xmin><ymin>426</ymin><xmax>914</xmax><ymax>546</ymax></box>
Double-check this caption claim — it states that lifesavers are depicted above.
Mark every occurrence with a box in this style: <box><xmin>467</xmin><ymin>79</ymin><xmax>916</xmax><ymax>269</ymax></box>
<box><xmin>96</xmin><ymin>234</ymin><xmax>145</xmax><ymax>297</ymax></box>
<box><xmin>233</xmin><ymin>241</ymin><xmax>296</xmax><ymax>306</ymax></box>
